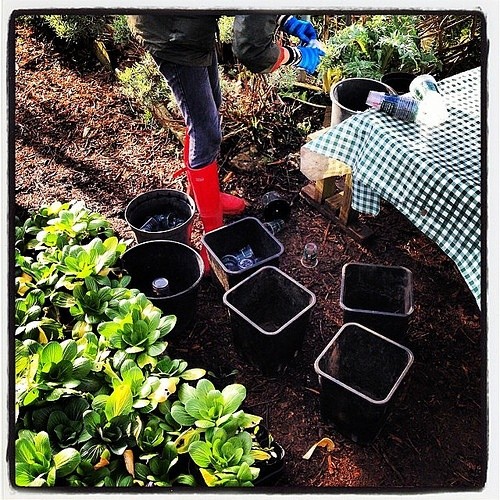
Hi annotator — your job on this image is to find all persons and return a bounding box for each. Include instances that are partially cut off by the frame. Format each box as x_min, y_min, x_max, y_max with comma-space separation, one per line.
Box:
125, 13, 326, 273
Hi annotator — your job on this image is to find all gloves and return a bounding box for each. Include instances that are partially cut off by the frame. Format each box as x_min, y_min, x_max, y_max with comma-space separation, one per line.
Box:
278, 15, 316, 44
296, 46, 325, 75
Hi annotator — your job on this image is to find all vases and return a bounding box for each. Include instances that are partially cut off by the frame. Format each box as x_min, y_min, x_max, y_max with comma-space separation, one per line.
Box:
201, 216, 285, 298
125, 189, 195, 247
312, 322, 414, 423
339, 263, 414, 340
118, 240, 204, 330
222, 265, 316, 375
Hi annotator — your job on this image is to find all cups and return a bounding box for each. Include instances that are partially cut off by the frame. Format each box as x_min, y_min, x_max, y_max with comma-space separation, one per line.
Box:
263, 220, 285, 236
140, 212, 177, 231
300, 243, 319, 268
366, 75, 448, 126
222, 245, 258, 271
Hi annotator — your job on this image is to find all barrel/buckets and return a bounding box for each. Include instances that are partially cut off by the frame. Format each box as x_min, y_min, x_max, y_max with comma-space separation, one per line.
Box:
223, 265, 316, 362
313, 323, 414, 446
329, 77, 397, 127
201, 216, 284, 293
117, 239, 205, 341
340, 262, 414, 340
125, 189, 195, 246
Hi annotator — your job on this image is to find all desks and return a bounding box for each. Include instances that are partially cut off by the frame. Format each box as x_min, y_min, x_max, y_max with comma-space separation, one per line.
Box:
297, 65, 481, 311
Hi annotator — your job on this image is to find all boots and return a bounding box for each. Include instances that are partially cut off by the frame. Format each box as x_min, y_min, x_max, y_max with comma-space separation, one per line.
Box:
173, 130, 246, 215
184, 135, 225, 273
